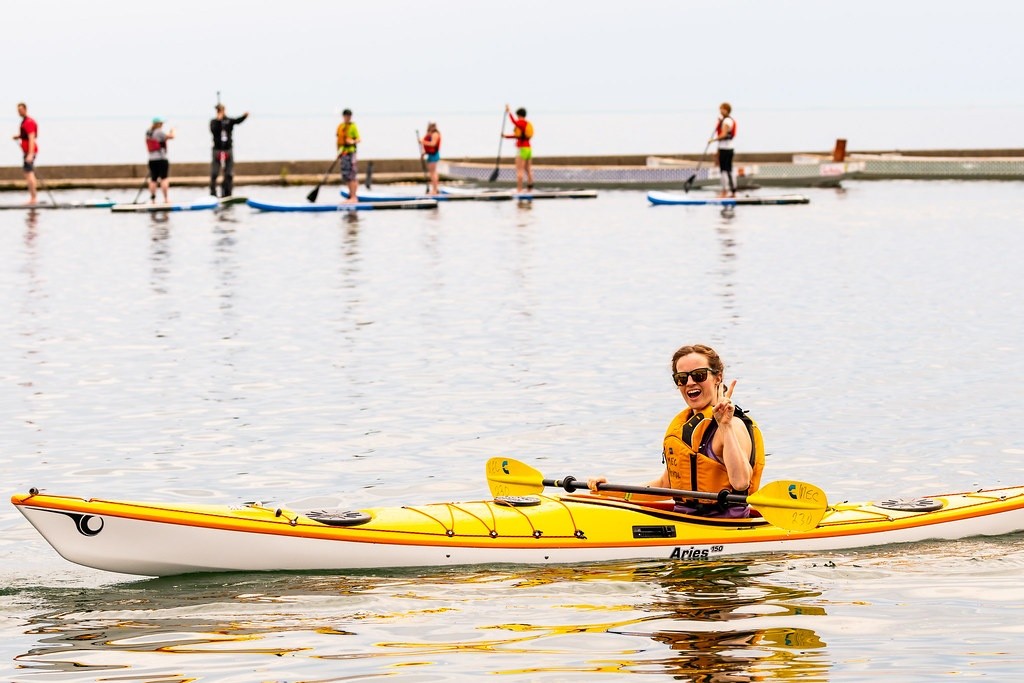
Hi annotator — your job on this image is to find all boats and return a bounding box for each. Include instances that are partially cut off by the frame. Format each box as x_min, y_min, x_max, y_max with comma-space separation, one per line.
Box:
12, 482, 1024, 579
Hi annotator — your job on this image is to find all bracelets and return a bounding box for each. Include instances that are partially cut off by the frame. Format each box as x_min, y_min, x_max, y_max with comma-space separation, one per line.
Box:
625, 492, 632, 501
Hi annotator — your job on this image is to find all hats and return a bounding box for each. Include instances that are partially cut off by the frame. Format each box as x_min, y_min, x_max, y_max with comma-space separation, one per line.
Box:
153, 117, 165, 124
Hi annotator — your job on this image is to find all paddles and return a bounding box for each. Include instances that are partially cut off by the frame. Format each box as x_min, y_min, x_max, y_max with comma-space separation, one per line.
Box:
488, 104, 509, 184
17, 139, 58, 206
216, 91, 232, 198
133, 170, 151, 204
485, 456, 829, 533
682, 127, 718, 194
307, 143, 348, 202
415, 129, 430, 193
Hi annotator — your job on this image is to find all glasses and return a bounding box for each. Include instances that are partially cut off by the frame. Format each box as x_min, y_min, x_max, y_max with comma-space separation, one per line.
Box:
673, 367, 718, 386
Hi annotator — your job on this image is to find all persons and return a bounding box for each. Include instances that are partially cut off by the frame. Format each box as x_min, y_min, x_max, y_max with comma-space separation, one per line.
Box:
708, 103, 736, 199
210, 103, 249, 199
418, 120, 441, 196
501, 104, 534, 193
586, 344, 755, 518
13, 104, 39, 205
336, 110, 359, 204
145, 117, 174, 205
